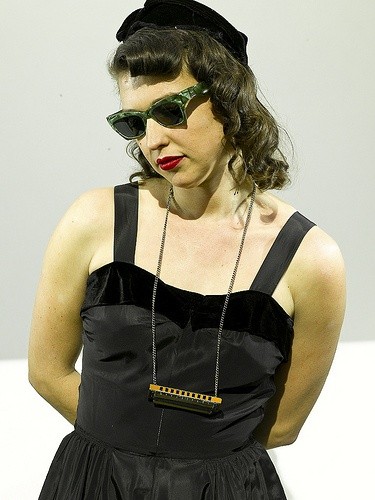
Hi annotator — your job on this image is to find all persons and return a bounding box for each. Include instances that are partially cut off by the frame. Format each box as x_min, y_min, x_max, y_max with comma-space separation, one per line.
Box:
28, 0, 345, 500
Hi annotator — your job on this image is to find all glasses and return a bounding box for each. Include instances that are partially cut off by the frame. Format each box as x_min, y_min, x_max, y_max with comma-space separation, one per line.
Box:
107, 83, 212, 141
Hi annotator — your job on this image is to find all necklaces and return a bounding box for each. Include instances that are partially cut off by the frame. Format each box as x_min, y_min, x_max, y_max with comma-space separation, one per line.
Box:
150, 182, 256, 413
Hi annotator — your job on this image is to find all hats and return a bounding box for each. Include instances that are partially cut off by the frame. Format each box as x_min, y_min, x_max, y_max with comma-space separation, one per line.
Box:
115, 0, 250, 67
